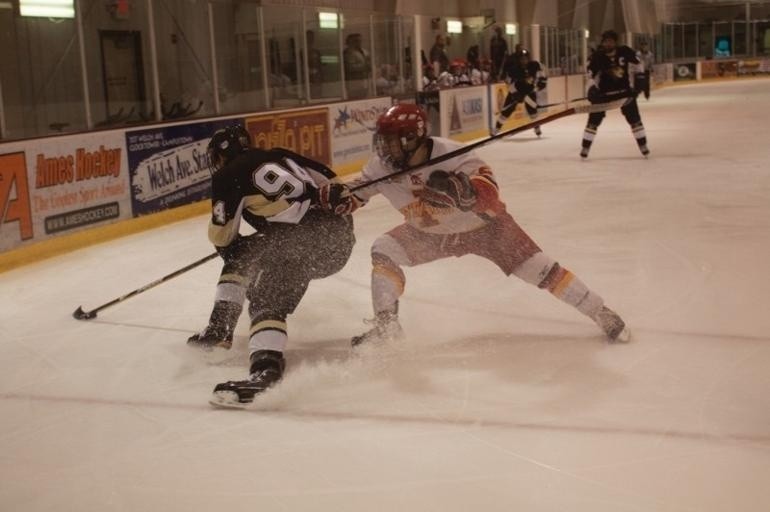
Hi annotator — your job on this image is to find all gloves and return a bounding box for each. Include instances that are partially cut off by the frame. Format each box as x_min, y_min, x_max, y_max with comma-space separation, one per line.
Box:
314, 183, 366, 217
420, 169, 478, 216
587, 84, 598, 101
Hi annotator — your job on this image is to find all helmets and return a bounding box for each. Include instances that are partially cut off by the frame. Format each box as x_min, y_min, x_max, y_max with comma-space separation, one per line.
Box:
600, 29, 618, 42
371, 102, 430, 170
517, 48, 530, 58
202, 119, 254, 180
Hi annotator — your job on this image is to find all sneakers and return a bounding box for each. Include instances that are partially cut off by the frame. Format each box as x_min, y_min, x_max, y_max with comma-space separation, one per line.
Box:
535, 125, 542, 135
590, 305, 628, 345
638, 143, 650, 155
350, 308, 404, 349
579, 146, 589, 158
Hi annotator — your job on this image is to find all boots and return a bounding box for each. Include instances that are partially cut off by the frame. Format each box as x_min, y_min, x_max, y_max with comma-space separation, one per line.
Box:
184, 297, 242, 351
211, 359, 285, 405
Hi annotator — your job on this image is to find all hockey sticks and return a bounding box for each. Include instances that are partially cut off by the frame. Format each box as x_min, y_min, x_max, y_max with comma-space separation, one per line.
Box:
95, 94, 203, 125
352, 88, 639, 192
73, 229, 262, 319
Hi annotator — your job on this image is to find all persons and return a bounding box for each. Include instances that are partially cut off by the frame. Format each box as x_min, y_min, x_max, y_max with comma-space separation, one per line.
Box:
313, 101, 629, 346
634, 41, 655, 101
183, 118, 355, 412
287, 23, 540, 93
488, 47, 549, 140
579, 29, 652, 162
